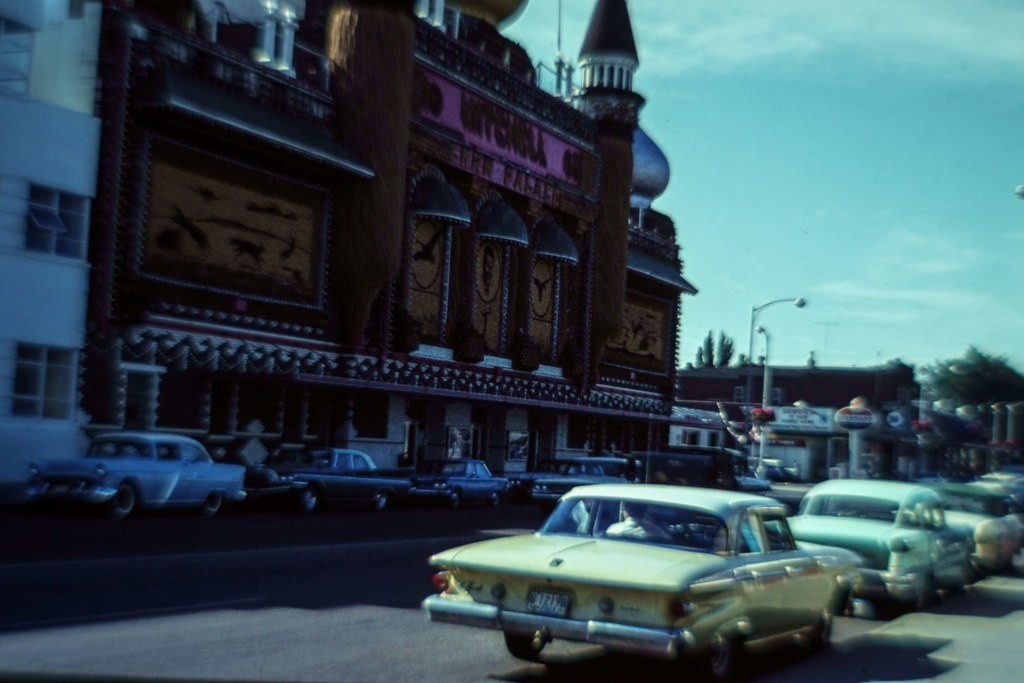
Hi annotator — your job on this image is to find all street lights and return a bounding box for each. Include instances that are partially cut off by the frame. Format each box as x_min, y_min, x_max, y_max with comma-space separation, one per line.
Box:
755, 324, 771, 479
746, 296, 807, 401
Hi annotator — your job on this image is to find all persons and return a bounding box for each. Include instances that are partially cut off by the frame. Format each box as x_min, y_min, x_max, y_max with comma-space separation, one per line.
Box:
604, 500, 674, 541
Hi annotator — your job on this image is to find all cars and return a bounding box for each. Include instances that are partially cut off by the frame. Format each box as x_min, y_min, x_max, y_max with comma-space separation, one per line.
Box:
420, 487, 864, 677
928, 481, 1024, 578
779, 477, 975, 620
402, 457, 508, 510
25, 433, 245, 519
245, 447, 416, 518
529, 457, 646, 507
